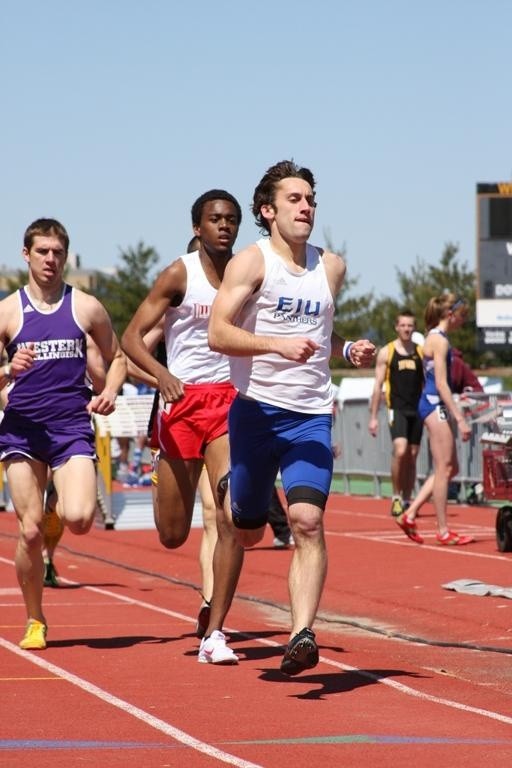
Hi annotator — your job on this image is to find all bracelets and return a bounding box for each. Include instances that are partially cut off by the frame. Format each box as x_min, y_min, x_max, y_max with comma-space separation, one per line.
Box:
7, 362, 12, 377
344, 340, 354, 365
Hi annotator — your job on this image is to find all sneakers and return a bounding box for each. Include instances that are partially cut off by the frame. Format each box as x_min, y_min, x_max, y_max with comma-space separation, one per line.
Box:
18, 619, 48, 649
396, 511, 424, 544
198, 630, 239, 665
280, 628, 318, 676
43, 489, 60, 536
195, 603, 211, 638
435, 532, 474, 546
150, 447, 160, 473
216, 469, 232, 506
273, 533, 296, 545
392, 497, 411, 515
43, 559, 58, 586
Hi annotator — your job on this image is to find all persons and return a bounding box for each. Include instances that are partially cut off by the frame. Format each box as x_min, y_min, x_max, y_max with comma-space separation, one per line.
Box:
370, 310, 426, 518
128, 237, 218, 636
121, 191, 246, 663
266, 481, 296, 549
0, 331, 109, 586
396, 289, 474, 544
0, 217, 130, 648
207, 161, 378, 673
